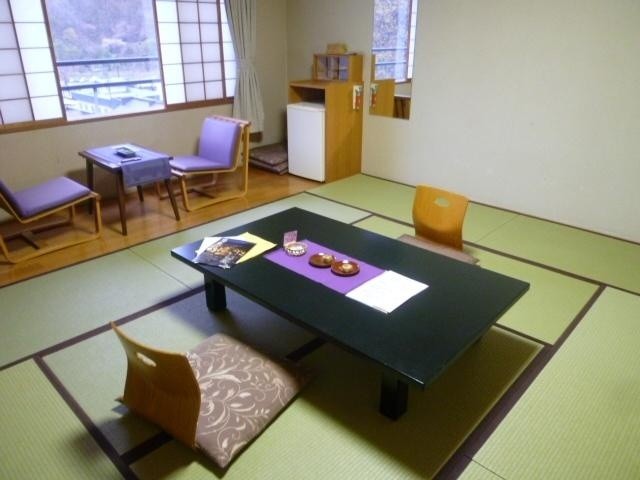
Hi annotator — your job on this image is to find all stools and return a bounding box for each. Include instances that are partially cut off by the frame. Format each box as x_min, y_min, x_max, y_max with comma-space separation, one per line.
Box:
412, 185, 469, 252
109, 319, 202, 453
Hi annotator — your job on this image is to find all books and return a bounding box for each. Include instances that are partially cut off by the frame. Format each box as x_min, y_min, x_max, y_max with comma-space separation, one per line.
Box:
196, 238, 256, 271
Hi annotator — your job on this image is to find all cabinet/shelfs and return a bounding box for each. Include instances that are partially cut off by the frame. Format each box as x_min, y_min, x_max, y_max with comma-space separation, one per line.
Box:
286, 43, 364, 183
370, 54, 395, 118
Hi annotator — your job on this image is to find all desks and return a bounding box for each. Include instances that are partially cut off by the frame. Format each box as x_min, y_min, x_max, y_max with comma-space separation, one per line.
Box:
170, 207, 531, 420
78, 143, 181, 235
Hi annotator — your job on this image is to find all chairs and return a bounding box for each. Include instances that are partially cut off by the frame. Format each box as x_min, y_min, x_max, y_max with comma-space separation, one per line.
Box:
155, 115, 251, 212
0, 176, 104, 265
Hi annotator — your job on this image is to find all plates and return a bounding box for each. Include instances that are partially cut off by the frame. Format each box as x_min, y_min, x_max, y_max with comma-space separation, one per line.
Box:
309, 253, 335, 268
332, 259, 361, 276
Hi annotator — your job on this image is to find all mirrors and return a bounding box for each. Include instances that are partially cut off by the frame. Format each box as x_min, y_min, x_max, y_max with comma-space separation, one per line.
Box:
368, 1, 418, 120
